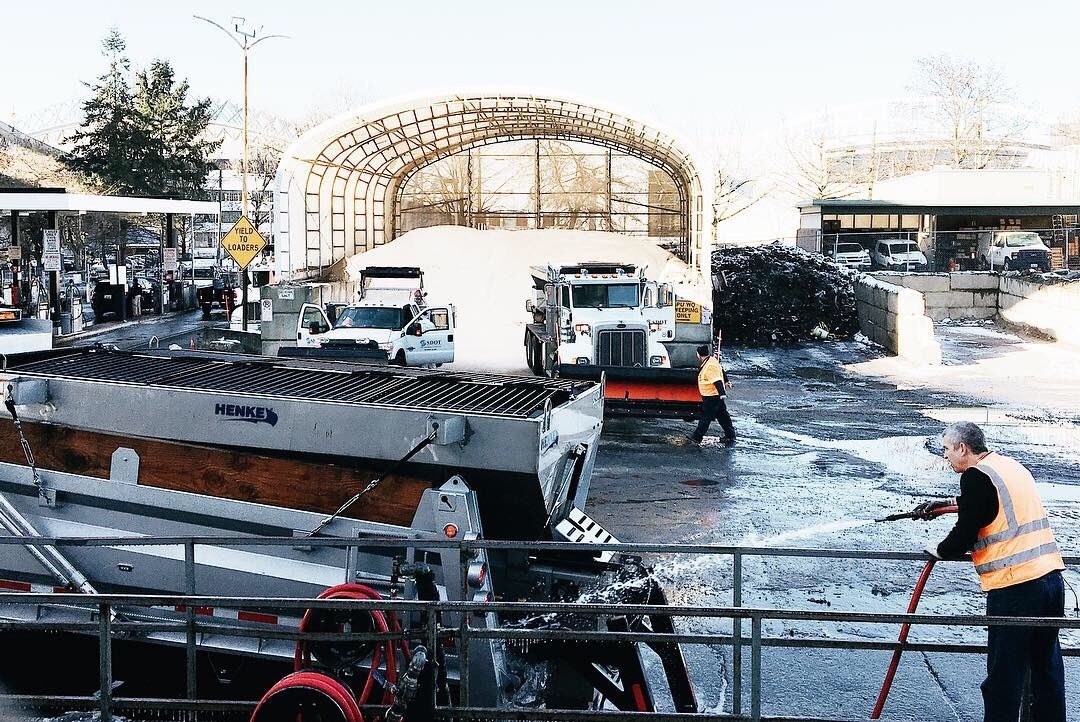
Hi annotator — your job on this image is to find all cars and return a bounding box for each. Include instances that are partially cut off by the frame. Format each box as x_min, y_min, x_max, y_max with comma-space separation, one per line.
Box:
90, 277, 159, 318
60, 281, 87, 300
825, 242, 872, 270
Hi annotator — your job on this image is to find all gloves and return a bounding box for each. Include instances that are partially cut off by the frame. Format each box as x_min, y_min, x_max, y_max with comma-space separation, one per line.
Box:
911, 498, 956, 521
922, 545, 943, 560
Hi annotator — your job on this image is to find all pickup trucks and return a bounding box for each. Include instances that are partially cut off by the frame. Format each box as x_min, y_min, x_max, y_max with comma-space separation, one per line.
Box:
195, 272, 244, 317
867, 238, 928, 274
295, 298, 457, 368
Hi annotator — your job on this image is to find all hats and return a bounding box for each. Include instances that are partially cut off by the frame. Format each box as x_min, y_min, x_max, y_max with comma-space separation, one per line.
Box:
697, 345, 709, 356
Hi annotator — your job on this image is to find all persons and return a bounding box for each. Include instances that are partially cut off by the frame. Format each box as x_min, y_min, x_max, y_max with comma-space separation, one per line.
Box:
131, 277, 142, 316
416, 312, 436, 369
685, 346, 735, 444
913, 420, 1067, 722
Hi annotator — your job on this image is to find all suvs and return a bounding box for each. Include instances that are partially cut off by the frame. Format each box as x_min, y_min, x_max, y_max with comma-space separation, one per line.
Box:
974, 230, 1053, 275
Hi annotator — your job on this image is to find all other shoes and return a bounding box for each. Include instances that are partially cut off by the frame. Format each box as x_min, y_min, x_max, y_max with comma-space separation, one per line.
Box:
719, 436, 735, 443
685, 433, 700, 443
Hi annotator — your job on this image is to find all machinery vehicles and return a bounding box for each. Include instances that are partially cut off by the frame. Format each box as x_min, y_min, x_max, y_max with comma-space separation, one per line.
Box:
521, 260, 719, 423
